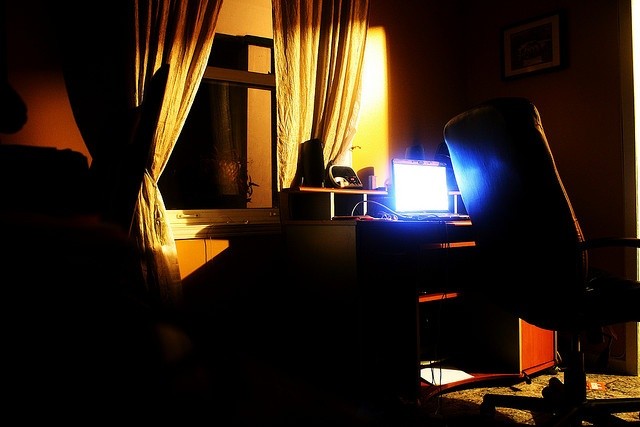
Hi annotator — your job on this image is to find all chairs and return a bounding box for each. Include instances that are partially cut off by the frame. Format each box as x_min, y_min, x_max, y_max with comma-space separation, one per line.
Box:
443, 99, 640, 427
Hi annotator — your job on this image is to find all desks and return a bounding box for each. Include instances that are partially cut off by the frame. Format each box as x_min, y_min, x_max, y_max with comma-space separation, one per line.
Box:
281, 219, 558, 427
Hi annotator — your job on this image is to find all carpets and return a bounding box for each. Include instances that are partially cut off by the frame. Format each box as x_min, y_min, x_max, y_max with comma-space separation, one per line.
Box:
419, 373, 640, 427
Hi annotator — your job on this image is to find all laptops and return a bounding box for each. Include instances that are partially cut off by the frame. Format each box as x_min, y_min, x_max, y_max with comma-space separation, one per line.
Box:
393, 158, 469, 223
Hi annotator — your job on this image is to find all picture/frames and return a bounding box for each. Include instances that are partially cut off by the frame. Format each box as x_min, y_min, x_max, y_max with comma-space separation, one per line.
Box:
498, 14, 570, 82
